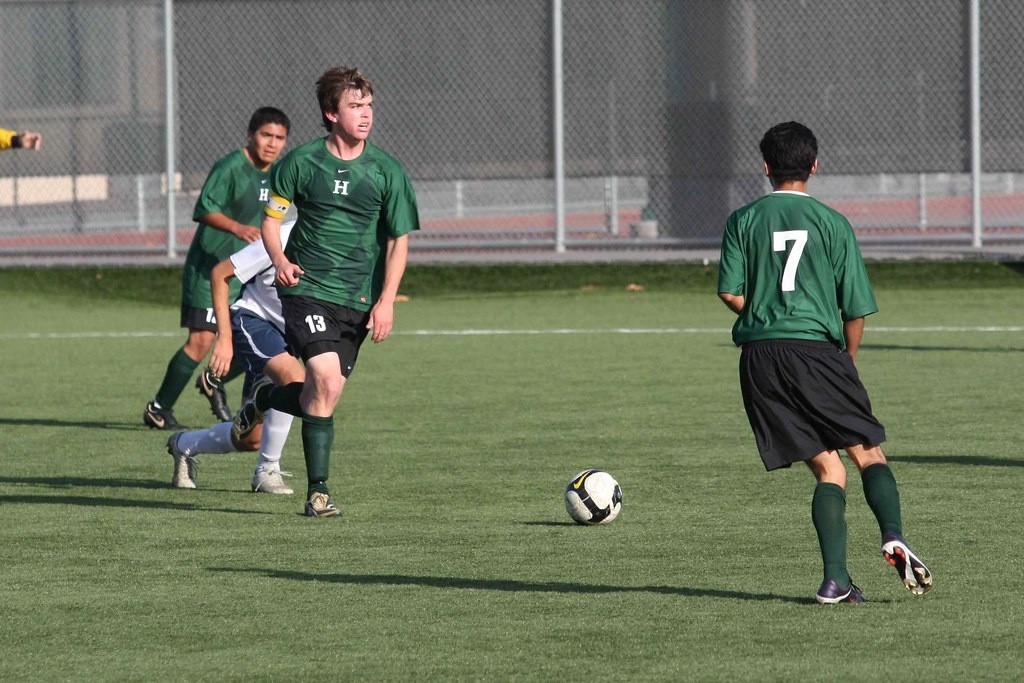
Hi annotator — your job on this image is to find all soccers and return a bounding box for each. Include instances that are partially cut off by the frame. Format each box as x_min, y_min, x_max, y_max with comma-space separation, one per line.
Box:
565, 468, 622, 525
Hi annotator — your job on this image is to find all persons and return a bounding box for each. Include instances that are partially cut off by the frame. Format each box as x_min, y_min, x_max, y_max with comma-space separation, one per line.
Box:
1, 119, 44, 157
237, 63, 423, 521
141, 105, 293, 433
164, 217, 310, 496
714, 121, 934, 603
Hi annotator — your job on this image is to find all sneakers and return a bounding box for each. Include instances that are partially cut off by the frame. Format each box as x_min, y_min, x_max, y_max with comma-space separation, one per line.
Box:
304, 491, 343, 518
250, 467, 294, 495
143, 401, 190, 431
195, 368, 233, 422
881, 532, 934, 595
166, 430, 197, 489
815, 578, 864, 604
